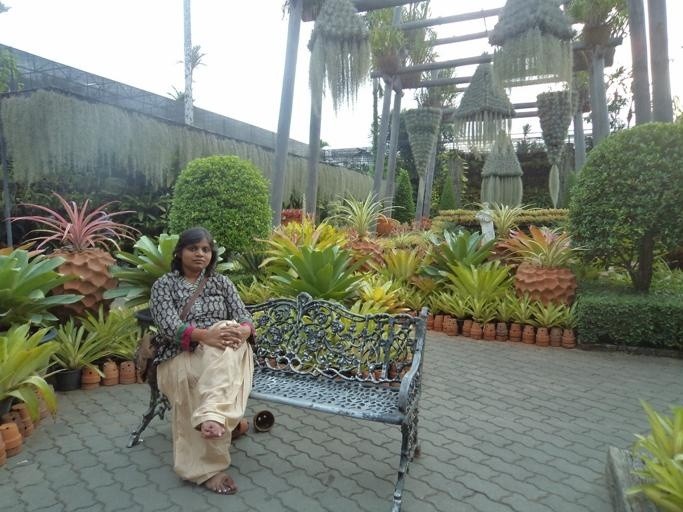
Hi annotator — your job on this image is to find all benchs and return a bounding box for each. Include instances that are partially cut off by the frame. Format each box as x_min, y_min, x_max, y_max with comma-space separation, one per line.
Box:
127, 290, 432, 512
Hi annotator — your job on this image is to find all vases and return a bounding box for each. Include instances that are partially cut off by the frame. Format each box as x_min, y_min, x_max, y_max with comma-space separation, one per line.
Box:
229, 409, 274, 439
0, 359, 147, 466
406, 309, 577, 348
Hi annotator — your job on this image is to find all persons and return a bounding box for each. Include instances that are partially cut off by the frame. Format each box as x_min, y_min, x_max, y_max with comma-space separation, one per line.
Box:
148, 226, 257, 496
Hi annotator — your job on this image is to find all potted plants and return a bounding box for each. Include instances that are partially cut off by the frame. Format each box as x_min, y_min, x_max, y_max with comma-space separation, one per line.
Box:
3, 189, 146, 322
99, 233, 225, 335
494, 224, 589, 311
323, 187, 405, 274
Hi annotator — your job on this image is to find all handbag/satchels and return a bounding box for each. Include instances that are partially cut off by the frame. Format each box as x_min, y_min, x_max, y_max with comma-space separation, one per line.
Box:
137, 332, 155, 382
